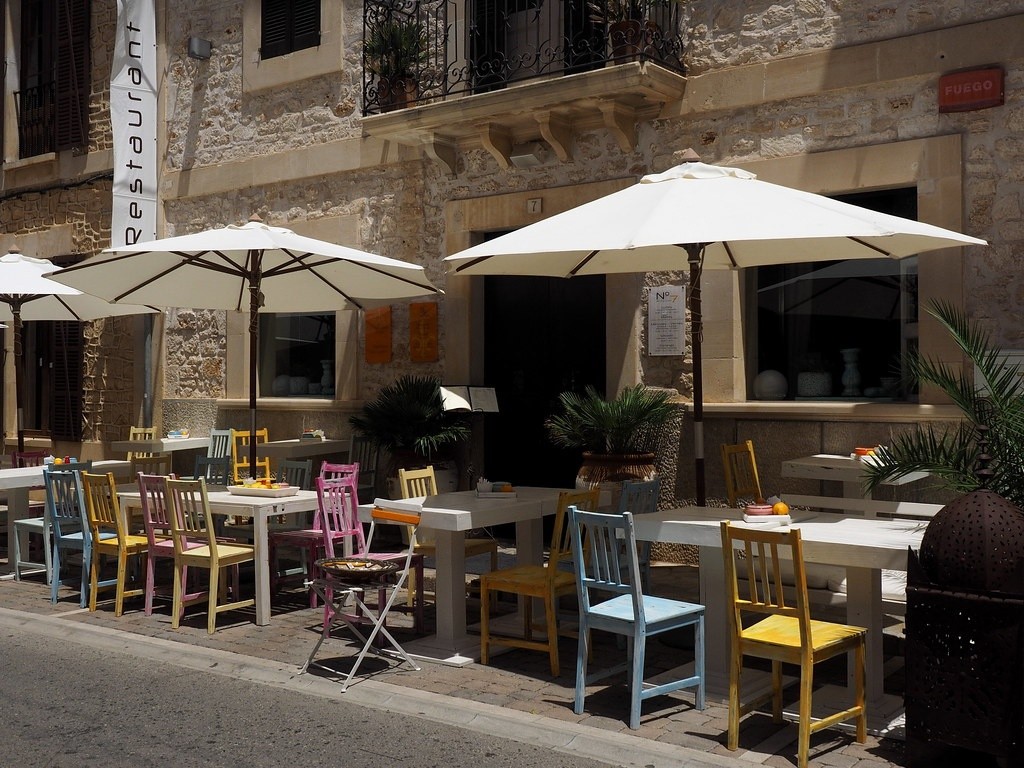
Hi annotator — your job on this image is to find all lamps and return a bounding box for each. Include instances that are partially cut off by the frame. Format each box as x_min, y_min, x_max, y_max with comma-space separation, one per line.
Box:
188, 37, 212, 61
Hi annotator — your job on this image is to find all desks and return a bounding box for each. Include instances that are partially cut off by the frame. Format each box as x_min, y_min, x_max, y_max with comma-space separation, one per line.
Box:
64, 459, 132, 568
70, 485, 353, 626
442, 487, 611, 642
0, 458, 62, 581
751, 514, 930, 742
239, 436, 351, 485
110, 433, 211, 475
615, 505, 817, 709
357, 486, 542, 667
782, 453, 932, 516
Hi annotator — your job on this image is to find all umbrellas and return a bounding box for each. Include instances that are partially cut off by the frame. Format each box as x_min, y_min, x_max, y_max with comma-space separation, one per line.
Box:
40, 215, 446, 480
441, 147, 989, 505
0, 246, 166, 469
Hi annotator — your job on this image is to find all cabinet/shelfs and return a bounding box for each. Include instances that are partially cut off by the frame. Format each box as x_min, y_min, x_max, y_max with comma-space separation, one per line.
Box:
900, 256, 918, 402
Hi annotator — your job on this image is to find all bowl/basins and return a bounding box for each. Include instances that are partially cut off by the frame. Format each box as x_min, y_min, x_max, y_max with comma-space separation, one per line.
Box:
855, 448, 873, 456
274, 483, 289, 488
493, 482, 513, 492
746, 505, 773, 516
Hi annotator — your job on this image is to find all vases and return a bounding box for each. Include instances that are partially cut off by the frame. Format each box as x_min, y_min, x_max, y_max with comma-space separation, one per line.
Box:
839, 347, 864, 396
320, 360, 335, 395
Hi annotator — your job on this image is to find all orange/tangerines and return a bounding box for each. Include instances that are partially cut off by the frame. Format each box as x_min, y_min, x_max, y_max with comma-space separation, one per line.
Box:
773, 502, 789, 515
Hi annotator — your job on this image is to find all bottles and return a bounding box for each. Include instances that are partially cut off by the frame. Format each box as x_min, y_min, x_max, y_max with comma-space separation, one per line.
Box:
767, 494, 781, 506
841, 350, 862, 397
65, 455, 70, 464
44, 455, 55, 465
758, 498, 764, 505
69, 458, 77, 464
243, 477, 256, 486
874, 444, 888, 456
55, 458, 62, 464
170, 429, 188, 435
477, 478, 492, 493
305, 429, 324, 437
62, 457, 65, 464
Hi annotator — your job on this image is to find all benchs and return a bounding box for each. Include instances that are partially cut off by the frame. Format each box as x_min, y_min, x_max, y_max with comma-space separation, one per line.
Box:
735, 494, 947, 616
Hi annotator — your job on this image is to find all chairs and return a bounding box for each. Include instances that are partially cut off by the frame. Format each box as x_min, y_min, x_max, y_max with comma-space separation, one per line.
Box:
310, 433, 381, 504
179, 426, 233, 486
568, 505, 707, 731
162, 475, 257, 634
193, 454, 230, 488
13, 460, 92, 587
720, 439, 762, 506
137, 472, 243, 623
42, 467, 141, 609
129, 454, 172, 482
80, 470, 168, 617
554, 475, 660, 650
316, 474, 424, 647
478, 486, 602, 678
127, 425, 157, 461
301, 498, 423, 693
270, 460, 360, 610
230, 427, 271, 486
222, 458, 312, 590
719, 518, 870, 768
398, 465, 497, 617
11, 450, 50, 561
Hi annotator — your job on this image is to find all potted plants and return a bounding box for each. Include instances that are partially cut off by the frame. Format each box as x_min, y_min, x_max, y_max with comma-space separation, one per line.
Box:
588, 0, 672, 67
344, 375, 479, 547
544, 384, 678, 515
866, 296, 1024, 592
363, 23, 430, 114
